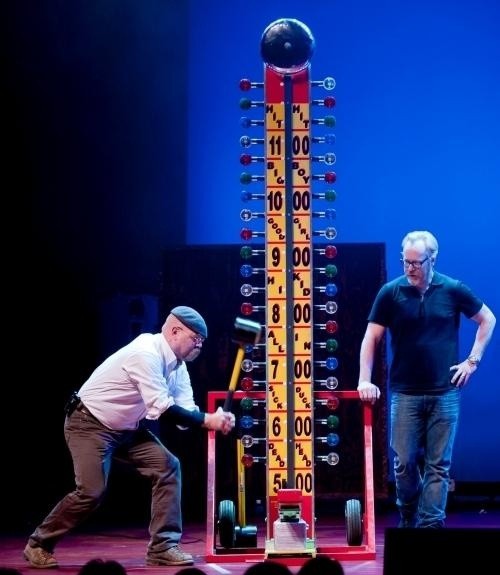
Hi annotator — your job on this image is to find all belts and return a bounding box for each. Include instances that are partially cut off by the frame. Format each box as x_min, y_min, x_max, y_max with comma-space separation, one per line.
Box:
77, 402, 91, 416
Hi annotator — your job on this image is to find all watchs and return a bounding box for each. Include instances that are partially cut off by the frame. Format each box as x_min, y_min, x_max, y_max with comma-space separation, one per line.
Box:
467, 354, 480, 366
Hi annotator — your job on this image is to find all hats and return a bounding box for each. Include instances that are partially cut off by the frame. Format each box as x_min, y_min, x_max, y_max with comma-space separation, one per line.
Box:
170, 305, 208, 339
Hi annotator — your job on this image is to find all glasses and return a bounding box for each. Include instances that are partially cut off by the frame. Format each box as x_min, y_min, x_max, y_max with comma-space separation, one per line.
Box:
177, 326, 206, 343
401, 259, 429, 269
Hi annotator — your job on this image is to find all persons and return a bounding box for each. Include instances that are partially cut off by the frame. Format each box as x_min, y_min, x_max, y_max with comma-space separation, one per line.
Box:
21, 304, 239, 566
79, 558, 127, 575
245, 555, 344, 575
356, 228, 498, 530
176, 568, 205, 575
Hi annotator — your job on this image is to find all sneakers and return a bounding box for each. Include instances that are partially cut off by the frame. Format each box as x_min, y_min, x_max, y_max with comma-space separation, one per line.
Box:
146, 547, 195, 566
23, 542, 60, 568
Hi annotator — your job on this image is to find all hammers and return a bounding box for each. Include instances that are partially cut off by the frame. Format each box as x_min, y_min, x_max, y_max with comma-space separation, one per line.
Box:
223, 335, 254, 412
